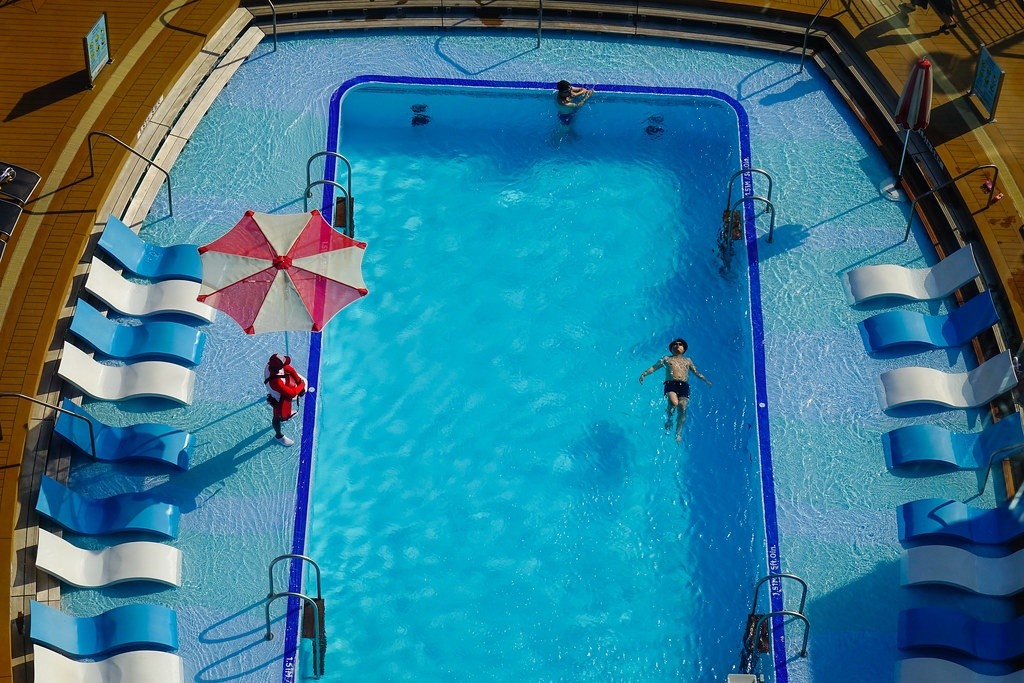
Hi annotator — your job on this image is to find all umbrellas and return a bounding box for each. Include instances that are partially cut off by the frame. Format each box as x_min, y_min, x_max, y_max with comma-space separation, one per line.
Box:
196, 208, 370, 366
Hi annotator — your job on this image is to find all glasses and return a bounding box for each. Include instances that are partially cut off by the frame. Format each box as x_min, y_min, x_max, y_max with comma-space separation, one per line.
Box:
674, 343, 683, 346
562, 87, 572, 94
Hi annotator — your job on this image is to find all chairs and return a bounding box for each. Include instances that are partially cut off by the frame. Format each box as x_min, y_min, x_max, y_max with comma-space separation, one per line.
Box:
895, 480, 1024, 541
843, 242, 980, 307
33, 527, 186, 590
877, 349, 1019, 415
55, 340, 199, 408
892, 656, 1024, 682
900, 544, 1024, 596
52, 397, 197, 473
855, 286, 1002, 358
896, 606, 1024, 654
29, 596, 182, 656
84, 256, 222, 325
31, 642, 185, 683
879, 411, 1024, 472
96, 213, 228, 292
33, 474, 182, 540
67, 297, 209, 367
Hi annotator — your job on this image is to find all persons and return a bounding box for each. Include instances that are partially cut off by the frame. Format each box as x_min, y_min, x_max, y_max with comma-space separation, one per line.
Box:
556, 80, 594, 141
263, 353, 304, 448
639, 338, 714, 440
718, 211, 741, 272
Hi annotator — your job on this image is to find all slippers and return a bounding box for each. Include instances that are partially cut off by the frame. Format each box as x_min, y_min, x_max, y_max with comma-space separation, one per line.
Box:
273, 434, 294, 447
281, 410, 298, 423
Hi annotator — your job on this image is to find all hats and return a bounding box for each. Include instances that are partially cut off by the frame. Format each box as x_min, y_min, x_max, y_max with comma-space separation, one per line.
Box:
268, 353, 291, 367
